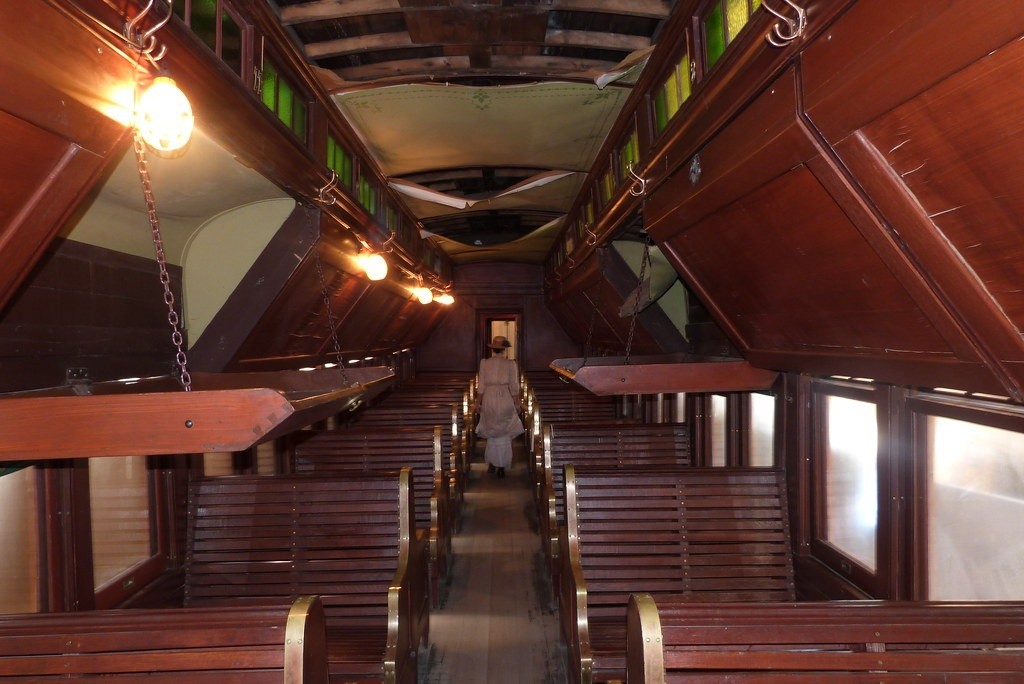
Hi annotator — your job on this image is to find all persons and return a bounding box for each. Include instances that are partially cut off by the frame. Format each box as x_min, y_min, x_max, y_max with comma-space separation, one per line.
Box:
475, 336, 524, 478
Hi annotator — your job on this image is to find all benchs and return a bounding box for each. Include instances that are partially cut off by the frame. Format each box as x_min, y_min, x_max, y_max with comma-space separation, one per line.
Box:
0, 368, 480, 683
521, 370, 1024, 683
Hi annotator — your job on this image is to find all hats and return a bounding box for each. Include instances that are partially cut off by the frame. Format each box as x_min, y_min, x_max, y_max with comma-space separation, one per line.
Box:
487, 336, 512, 348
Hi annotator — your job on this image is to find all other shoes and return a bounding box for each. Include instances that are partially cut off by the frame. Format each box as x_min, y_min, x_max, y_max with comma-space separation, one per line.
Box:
497, 468, 505, 477
488, 463, 495, 472
